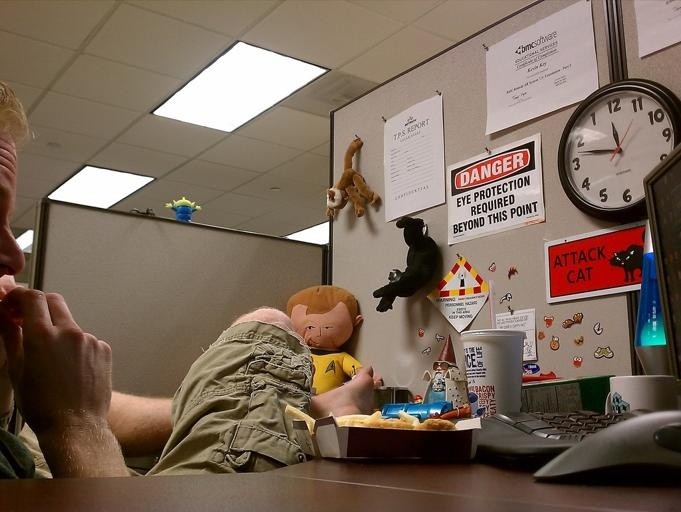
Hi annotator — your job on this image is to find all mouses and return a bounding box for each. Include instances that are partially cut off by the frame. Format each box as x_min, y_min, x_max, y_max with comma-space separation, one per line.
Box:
533, 409, 681, 489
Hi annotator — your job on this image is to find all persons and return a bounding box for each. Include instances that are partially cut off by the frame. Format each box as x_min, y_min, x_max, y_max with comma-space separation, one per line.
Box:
1, 78, 383, 481
283, 286, 382, 398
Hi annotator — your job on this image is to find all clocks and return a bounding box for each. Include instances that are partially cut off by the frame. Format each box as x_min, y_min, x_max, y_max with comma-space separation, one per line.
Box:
557, 78, 681, 224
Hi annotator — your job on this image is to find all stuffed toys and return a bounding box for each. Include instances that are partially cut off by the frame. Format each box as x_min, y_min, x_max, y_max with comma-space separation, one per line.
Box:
371, 215, 441, 312
324, 138, 379, 219
163, 196, 203, 222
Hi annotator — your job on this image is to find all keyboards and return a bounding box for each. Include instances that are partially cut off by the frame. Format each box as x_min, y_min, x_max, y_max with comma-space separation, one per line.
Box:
477, 411, 639, 474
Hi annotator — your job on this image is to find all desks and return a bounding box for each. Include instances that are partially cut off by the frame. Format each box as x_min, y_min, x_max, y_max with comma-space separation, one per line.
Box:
0, 456, 681, 512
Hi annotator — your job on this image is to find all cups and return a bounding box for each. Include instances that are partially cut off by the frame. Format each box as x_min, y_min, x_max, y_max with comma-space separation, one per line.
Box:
460, 328, 526, 421
609, 373, 681, 416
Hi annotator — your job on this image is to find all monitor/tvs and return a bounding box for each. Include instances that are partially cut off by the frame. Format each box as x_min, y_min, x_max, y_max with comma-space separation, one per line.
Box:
643, 144, 681, 388
